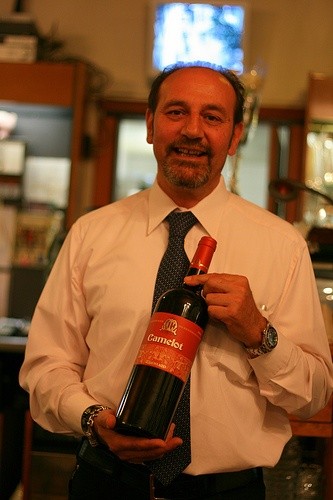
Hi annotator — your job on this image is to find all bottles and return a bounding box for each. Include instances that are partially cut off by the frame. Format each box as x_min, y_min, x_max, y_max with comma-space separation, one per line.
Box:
114, 236, 217, 441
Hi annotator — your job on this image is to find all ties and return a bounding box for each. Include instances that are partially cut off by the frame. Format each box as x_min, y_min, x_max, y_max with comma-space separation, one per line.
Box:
145, 208, 197, 489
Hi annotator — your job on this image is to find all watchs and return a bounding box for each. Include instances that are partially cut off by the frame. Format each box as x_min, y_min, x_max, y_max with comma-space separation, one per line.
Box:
242, 317, 278, 357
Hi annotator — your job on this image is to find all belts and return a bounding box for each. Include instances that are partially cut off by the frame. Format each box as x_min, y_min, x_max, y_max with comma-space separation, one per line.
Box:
80, 442, 260, 499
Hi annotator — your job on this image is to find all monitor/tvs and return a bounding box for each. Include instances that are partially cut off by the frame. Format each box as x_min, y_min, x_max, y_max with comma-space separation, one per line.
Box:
145, 0, 250, 80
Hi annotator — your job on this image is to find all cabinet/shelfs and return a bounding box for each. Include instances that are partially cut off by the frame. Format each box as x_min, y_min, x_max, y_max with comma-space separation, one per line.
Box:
1, 61, 332, 500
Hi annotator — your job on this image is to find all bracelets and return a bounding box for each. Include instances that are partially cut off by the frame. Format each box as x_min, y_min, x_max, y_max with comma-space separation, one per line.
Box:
81, 405, 111, 447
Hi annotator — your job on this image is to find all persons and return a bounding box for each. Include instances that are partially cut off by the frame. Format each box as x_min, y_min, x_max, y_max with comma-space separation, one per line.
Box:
19, 61, 333, 500
